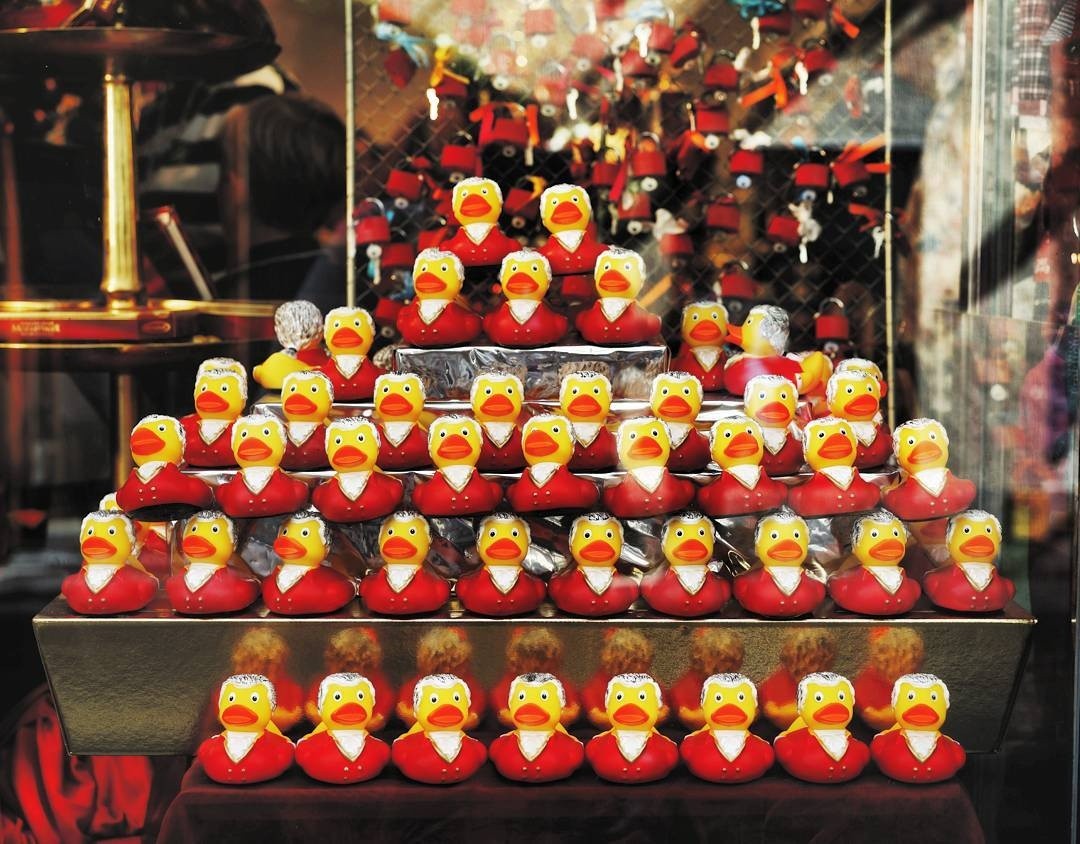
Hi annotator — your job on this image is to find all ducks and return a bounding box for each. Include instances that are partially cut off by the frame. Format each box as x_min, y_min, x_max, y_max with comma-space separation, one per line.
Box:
61, 177, 1017, 784
